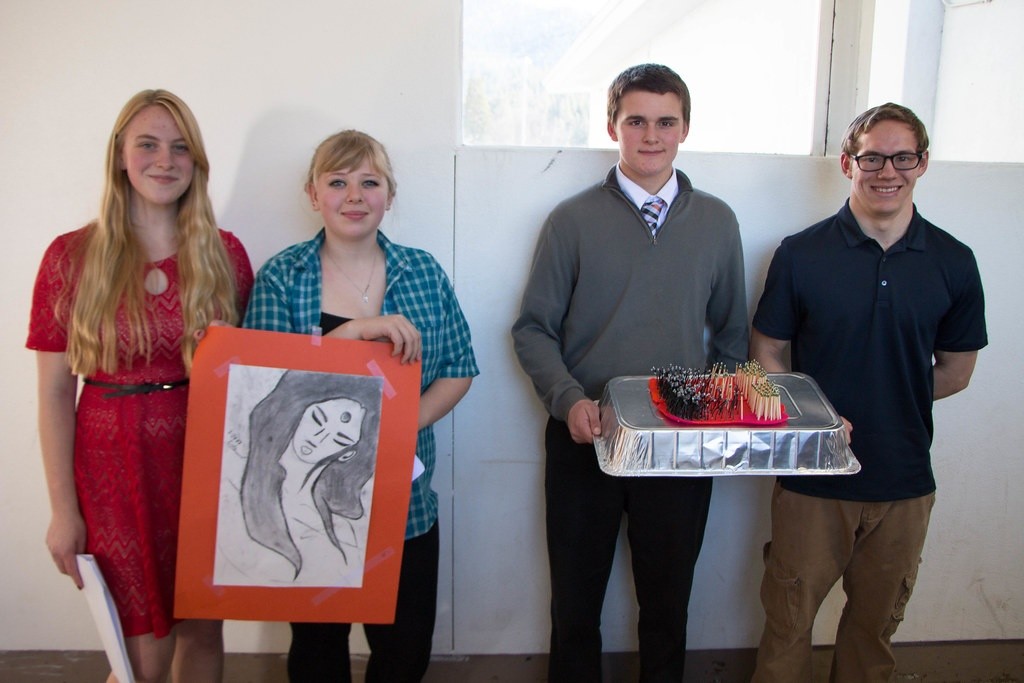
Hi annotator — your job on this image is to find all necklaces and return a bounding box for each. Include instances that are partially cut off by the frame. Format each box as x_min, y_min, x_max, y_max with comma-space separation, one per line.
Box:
320, 244, 380, 305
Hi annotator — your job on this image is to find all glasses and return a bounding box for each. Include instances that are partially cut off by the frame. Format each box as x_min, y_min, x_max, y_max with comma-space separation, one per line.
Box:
850, 152, 923, 172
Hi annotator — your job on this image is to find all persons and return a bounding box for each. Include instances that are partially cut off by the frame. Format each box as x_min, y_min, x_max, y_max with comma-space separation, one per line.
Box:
244, 129, 479, 683
25, 88, 255, 683
510, 63, 750, 683
751, 103, 988, 682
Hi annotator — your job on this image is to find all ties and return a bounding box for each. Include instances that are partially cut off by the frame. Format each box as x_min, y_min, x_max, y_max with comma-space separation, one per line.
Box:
641, 198, 665, 240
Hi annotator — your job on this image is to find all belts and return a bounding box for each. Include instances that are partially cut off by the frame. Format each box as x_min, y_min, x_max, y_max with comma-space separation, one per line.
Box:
83, 378, 190, 399
